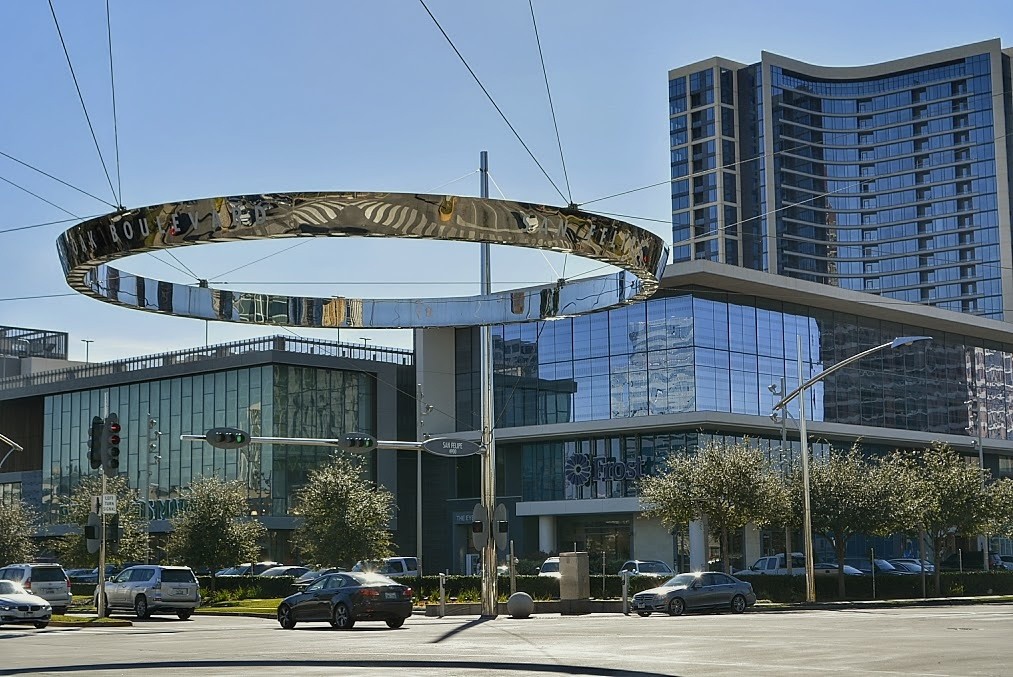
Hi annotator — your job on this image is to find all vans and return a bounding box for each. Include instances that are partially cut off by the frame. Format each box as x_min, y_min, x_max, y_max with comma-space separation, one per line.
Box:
351, 556, 419, 579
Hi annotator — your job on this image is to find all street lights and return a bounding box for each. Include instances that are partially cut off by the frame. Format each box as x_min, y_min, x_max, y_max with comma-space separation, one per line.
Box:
80, 339, 94, 366
772, 333, 933, 612
144, 413, 164, 566
767, 376, 793, 577
964, 397, 989, 571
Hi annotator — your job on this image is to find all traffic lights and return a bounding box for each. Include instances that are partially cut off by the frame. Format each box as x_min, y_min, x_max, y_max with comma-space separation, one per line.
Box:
338, 432, 378, 453
206, 427, 251, 450
87, 416, 104, 469
470, 502, 489, 551
100, 412, 121, 481
109, 513, 128, 557
84, 512, 99, 554
493, 503, 510, 551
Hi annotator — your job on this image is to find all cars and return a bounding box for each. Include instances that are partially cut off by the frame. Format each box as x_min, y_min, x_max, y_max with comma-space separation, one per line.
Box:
293, 567, 347, 592
208, 567, 231, 576
260, 565, 311, 579
998, 555, 1013, 571
0, 580, 54, 628
813, 563, 865, 578
276, 571, 413, 631
66, 569, 94, 577
12, 338, 31, 353
631, 573, 758, 618
618, 559, 674, 577
842, 558, 936, 574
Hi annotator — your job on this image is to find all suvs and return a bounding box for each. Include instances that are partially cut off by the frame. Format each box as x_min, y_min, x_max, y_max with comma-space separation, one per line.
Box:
535, 557, 561, 580
730, 552, 806, 576
940, 550, 1006, 571
224, 561, 284, 576
93, 565, 202, 621
0, 563, 72, 615
70, 562, 145, 596
